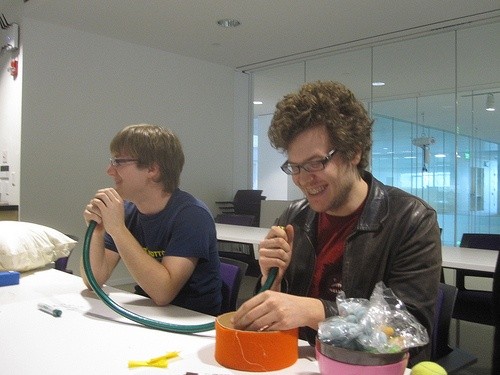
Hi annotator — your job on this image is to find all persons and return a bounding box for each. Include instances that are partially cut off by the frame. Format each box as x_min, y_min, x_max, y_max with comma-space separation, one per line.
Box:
230, 79, 442, 369
79, 124, 223, 317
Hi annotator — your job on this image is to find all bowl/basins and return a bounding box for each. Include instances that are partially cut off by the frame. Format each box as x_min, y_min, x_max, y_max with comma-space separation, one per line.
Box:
315, 335, 405, 366
315, 347, 409, 375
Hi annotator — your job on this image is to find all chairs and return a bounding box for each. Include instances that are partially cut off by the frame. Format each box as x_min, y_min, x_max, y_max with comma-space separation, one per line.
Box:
211, 189, 265, 228
218, 258, 249, 312
432, 234, 500, 375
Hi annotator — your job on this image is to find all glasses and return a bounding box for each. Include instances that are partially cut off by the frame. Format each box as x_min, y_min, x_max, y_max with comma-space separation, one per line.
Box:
281, 149, 338, 175
107, 158, 147, 168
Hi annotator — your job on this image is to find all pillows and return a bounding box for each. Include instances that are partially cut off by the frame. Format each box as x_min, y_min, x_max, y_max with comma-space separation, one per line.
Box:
0, 221, 80, 274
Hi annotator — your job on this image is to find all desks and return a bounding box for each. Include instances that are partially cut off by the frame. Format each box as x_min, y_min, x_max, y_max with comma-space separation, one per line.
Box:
214, 221, 269, 243
440, 245, 499, 274
0, 268, 414, 375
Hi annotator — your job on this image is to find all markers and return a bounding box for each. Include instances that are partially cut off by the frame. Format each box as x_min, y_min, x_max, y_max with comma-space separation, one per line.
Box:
36, 301, 63, 318
